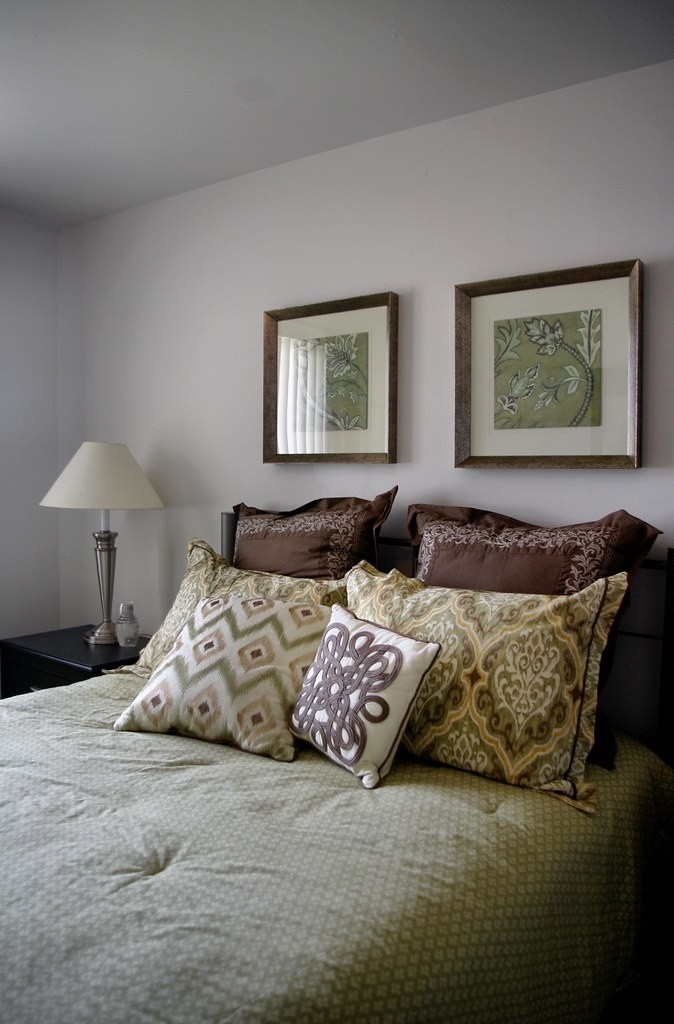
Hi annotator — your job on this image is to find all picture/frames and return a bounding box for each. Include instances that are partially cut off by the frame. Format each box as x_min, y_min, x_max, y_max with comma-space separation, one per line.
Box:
263, 291, 400, 465
453, 259, 642, 471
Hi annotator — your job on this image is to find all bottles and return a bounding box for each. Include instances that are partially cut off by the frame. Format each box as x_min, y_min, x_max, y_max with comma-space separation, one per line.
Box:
116, 601, 139, 648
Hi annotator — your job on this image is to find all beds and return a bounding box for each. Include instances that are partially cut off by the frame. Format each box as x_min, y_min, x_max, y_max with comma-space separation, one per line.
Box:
1, 512, 674, 1024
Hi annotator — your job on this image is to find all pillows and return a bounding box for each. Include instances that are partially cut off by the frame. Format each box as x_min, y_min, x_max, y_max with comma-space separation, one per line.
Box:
344, 560, 629, 813
115, 597, 331, 762
290, 602, 444, 790
102, 535, 348, 680
406, 504, 664, 693
233, 485, 399, 580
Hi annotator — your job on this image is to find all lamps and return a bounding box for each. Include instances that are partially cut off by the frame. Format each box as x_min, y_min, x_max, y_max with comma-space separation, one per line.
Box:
39, 441, 165, 645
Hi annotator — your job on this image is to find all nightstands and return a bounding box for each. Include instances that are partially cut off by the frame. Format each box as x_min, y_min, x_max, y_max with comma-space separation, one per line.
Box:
0, 624, 153, 700
